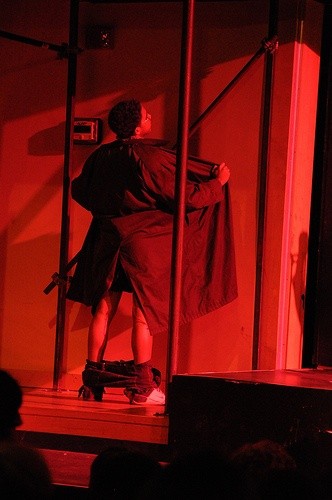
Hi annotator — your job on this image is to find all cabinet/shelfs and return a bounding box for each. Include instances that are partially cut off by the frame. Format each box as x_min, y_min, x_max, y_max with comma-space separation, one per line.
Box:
164, 365, 332, 500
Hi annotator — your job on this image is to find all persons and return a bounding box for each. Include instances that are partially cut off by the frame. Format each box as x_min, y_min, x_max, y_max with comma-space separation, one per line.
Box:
69, 97, 231, 405
0, 367, 331, 500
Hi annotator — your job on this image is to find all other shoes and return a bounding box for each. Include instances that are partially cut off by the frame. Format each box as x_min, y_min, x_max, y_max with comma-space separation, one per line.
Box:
133, 386, 167, 406
82, 385, 102, 399
